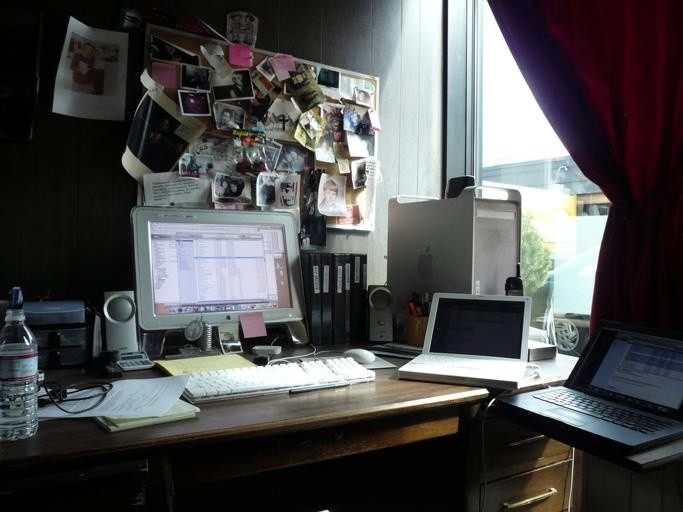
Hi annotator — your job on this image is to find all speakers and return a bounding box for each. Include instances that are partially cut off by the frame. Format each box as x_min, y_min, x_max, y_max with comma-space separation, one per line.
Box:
103, 291, 138, 354
367, 284, 394, 343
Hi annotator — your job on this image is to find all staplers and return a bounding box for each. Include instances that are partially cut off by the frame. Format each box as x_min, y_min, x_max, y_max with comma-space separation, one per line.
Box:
82, 351, 121, 379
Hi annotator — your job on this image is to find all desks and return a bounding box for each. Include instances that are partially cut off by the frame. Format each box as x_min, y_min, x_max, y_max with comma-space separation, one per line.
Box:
0, 339, 584, 510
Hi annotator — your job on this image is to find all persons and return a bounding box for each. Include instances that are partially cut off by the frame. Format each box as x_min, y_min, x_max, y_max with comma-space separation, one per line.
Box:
69, 41, 105, 96
216, 108, 240, 130
229, 70, 249, 98
203, 162, 215, 178
185, 155, 202, 178
319, 179, 345, 216
259, 175, 275, 205
278, 181, 297, 206
213, 174, 244, 199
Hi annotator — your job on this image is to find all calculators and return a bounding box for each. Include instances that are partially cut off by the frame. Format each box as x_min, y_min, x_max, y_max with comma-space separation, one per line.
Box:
117, 352, 155, 372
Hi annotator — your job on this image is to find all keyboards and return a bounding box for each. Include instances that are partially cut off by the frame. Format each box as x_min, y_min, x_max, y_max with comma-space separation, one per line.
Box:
172, 357, 376, 404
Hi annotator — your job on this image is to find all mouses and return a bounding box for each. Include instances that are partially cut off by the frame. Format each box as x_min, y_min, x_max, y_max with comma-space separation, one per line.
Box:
344, 348, 377, 364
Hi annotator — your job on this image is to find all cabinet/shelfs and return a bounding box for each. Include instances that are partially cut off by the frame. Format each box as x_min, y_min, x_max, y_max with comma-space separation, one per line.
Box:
457, 404, 583, 511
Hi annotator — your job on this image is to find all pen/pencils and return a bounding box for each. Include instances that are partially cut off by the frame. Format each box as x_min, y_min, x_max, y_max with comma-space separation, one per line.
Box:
408, 291, 433, 317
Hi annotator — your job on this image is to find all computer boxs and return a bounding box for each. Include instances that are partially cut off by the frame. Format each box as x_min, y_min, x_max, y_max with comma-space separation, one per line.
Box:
387, 185, 521, 344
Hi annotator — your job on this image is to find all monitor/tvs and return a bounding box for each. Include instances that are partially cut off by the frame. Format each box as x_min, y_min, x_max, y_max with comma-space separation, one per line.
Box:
130, 205, 307, 361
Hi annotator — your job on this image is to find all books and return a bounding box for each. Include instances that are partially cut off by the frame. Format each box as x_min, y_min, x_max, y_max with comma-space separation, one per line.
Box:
151, 352, 261, 376
92, 411, 196, 433
102, 398, 200, 427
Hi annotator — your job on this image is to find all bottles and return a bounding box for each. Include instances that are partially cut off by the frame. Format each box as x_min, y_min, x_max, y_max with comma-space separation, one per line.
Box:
0, 309, 38, 441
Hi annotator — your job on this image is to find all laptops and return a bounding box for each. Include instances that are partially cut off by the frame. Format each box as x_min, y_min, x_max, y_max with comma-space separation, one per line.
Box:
495, 319, 683, 455
397, 293, 532, 391
